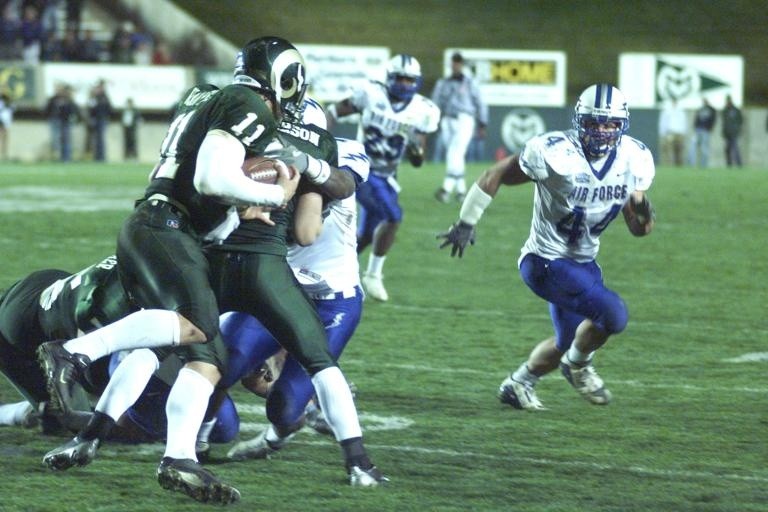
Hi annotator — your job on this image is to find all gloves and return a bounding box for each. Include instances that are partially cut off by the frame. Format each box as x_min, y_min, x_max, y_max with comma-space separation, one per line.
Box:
631, 194, 654, 233
436, 219, 475, 258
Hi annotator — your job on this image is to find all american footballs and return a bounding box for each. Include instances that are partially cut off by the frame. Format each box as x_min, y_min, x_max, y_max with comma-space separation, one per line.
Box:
214, 157, 295, 207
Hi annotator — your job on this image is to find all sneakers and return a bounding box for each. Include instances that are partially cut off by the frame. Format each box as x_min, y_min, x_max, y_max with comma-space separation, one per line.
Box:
362, 272, 388, 302
157, 456, 241, 508
559, 350, 613, 405
36, 340, 99, 473
347, 464, 391, 488
195, 402, 334, 459
497, 376, 550, 412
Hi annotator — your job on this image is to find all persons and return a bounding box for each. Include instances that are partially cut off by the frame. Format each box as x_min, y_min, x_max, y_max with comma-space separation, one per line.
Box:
499, 107, 545, 152
688, 98, 717, 171
660, 94, 689, 167
1, 1, 220, 66
430, 82, 657, 417
722, 92, 745, 168
428, 51, 492, 204
323, 54, 441, 304
0, 36, 388, 502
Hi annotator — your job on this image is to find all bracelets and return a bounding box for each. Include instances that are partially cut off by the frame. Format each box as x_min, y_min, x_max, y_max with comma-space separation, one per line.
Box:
454, 181, 492, 231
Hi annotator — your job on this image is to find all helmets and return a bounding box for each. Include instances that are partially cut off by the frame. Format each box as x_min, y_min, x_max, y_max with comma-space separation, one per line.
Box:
233, 36, 328, 130
573, 83, 630, 158
385, 54, 423, 100
